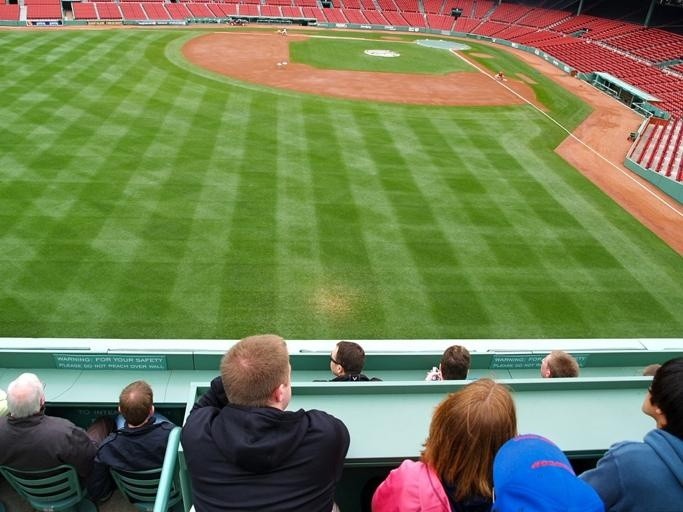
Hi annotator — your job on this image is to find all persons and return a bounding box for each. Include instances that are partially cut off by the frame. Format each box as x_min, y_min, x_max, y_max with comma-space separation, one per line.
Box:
0, 371, 119, 506
310, 337, 385, 382
574, 354, 682, 512
356, 377, 519, 512
537, 348, 580, 380
91, 379, 180, 505
181, 333, 351, 512
423, 343, 471, 380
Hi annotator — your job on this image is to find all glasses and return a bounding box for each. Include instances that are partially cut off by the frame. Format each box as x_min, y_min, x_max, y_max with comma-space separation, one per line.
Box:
327, 354, 339, 365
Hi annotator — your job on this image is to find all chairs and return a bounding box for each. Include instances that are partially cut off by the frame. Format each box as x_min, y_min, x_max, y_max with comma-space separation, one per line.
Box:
0, 464, 181, 512
0, 0, 525, 46
524, 2, 681, 186
